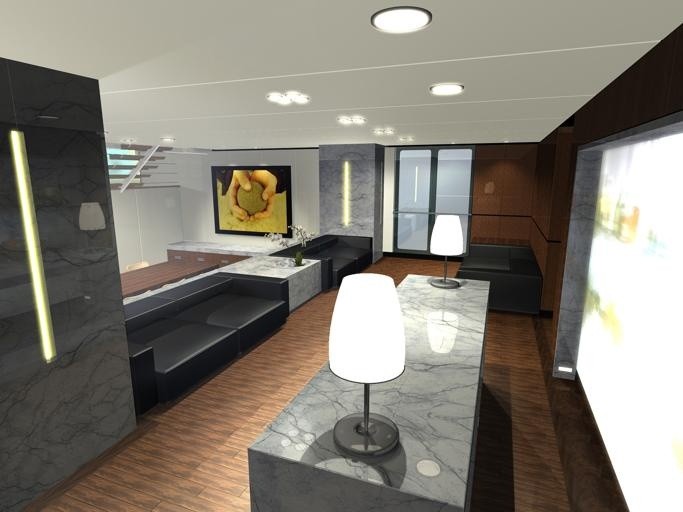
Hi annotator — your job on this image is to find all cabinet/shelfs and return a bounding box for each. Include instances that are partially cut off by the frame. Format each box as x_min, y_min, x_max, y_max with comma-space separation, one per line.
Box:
166, 250, 251, 266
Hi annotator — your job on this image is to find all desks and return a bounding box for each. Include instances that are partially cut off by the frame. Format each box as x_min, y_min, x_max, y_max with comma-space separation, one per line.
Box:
247, 274, 491, 511
120, 258, 219, 299
218, 254, 322, 313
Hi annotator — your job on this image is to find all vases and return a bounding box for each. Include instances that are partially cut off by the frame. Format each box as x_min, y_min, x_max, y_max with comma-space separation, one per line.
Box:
295, 252, 303, 265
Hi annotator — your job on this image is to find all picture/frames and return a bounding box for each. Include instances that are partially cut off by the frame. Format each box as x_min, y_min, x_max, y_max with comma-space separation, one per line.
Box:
210, 165, 293, 239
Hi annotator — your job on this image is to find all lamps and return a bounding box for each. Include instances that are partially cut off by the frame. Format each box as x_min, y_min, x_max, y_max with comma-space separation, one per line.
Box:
78, 200, 106, 255
327, 271, 406, 459
429, 214, 465, 290
427, 289, 460, 354
370, 5, 433, 34
428, 83, 464, 96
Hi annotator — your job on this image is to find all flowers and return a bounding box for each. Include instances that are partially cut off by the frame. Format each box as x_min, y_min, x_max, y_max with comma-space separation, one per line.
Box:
263, 224, 317, 253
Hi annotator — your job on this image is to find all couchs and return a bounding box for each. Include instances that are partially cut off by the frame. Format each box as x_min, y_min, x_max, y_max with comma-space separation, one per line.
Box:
123, 273, 289, 414
269, 235, 373, 291
454, 242, 543, 317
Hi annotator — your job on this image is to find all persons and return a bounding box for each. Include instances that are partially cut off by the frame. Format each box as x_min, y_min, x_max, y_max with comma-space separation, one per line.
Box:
226, 170, 277, 221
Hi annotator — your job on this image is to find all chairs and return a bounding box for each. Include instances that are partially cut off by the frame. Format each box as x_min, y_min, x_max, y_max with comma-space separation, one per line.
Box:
125, 261, 149, 273
162, 278, 185, 288
123, 289, 151, 301
